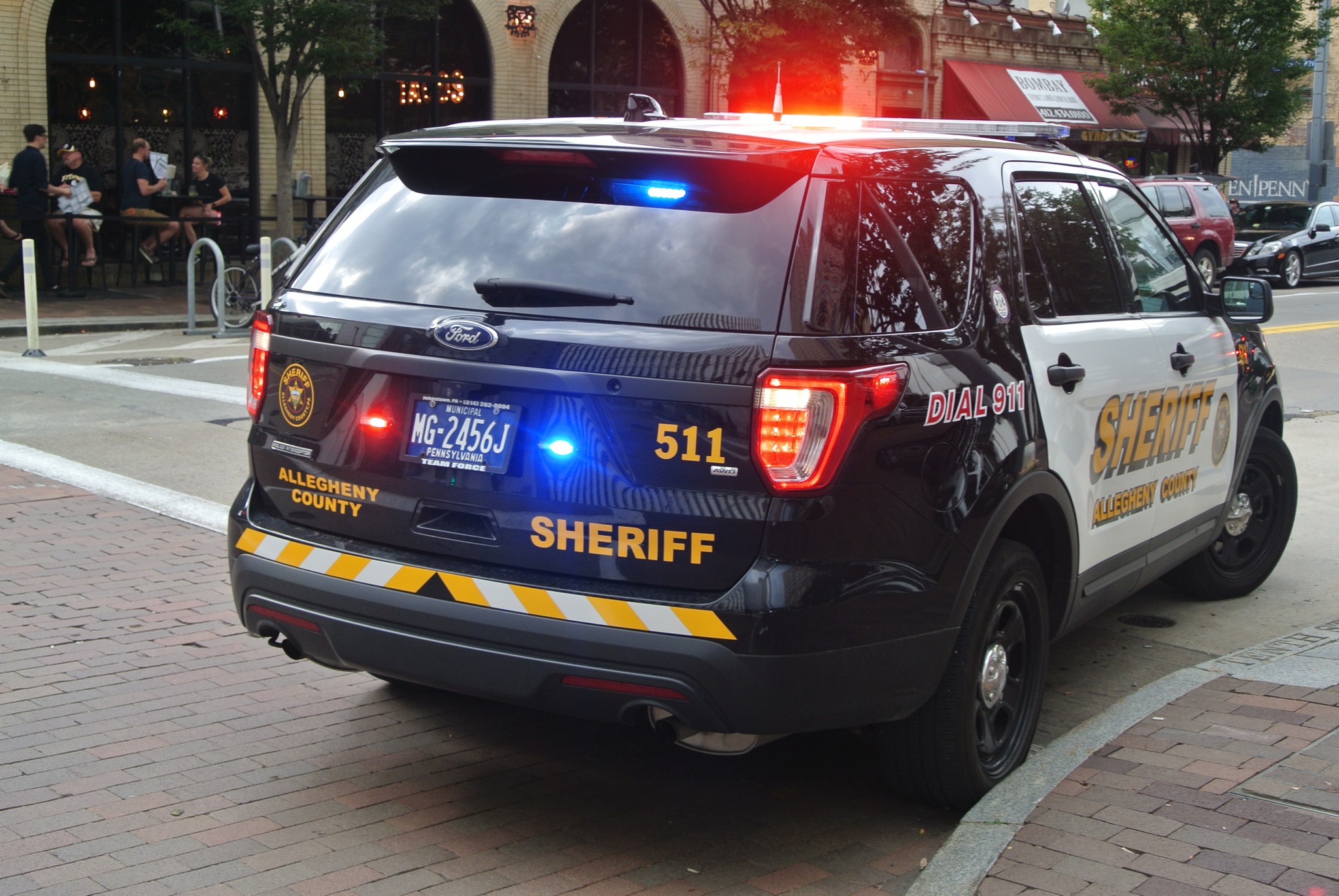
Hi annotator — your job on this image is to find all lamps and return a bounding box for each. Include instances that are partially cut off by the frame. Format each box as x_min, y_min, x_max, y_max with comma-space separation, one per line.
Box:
1006, 15, 1022, 32
504, 4, 538, 38
1047, 20, 1062, 37
963, 9, 980, 27
1086, 24, 1101, 38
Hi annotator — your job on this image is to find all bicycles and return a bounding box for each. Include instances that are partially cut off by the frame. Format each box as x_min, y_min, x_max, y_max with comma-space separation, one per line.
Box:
209, 241, 292, 331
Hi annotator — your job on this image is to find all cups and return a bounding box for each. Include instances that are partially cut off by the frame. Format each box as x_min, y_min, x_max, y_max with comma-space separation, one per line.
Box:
189, 185, 195, 195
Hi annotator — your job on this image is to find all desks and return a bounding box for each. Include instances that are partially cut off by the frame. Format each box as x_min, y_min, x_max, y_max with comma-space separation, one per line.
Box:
272, 192, 344, 240
151, 195, 248, 281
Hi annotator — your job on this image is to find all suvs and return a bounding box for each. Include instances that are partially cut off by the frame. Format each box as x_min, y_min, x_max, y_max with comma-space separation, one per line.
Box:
1124, 174, 1235, 292
226, 91, 1301, 808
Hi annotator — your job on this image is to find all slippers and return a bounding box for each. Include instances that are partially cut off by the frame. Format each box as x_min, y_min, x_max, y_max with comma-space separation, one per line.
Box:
60, 257, 69, 267
3, 231, 23, 241
81, 255, 99, 267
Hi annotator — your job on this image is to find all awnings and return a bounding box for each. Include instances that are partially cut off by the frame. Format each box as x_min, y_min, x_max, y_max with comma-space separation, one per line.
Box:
940, 57, 1261, 148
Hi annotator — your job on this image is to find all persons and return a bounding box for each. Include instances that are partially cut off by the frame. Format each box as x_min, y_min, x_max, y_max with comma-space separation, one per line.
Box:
1229, 198, 1244, 218
118, 138, 180, 264
0, 123, 70, 298
0, 142, 106, 269
179, 154, 233, 264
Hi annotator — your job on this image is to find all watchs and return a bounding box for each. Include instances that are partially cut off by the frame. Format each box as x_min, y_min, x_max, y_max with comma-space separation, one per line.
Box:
212, 202, 215, 209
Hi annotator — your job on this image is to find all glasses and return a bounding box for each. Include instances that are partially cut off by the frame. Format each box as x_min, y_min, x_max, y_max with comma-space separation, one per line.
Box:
39, 134, 49, 138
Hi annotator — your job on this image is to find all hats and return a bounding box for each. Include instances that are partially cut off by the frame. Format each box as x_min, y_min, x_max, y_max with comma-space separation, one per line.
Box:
57, 143, 81, 155
1230, 198, 1239, 203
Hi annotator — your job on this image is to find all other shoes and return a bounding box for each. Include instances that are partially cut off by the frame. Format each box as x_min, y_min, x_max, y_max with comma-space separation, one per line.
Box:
46, 286, 69, 295
194, 253, 201, 263
0, 283, 14, 298
139, 246, 154, 264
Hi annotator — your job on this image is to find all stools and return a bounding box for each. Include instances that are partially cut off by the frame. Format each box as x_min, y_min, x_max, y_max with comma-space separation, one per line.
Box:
57, 217, 224, 291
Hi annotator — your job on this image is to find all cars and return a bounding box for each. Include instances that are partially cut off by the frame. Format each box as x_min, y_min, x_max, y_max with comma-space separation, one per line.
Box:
1226, 200, 1339, 288
